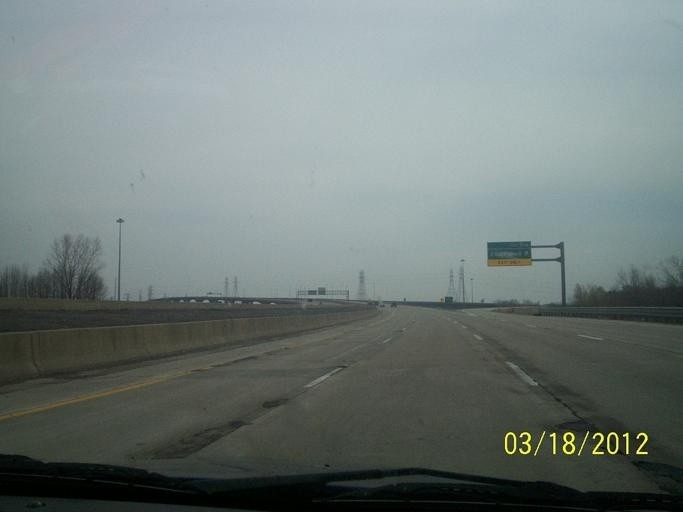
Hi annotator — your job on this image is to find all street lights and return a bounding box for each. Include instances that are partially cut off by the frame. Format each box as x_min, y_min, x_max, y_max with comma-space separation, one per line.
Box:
115, 217, 123, 301
